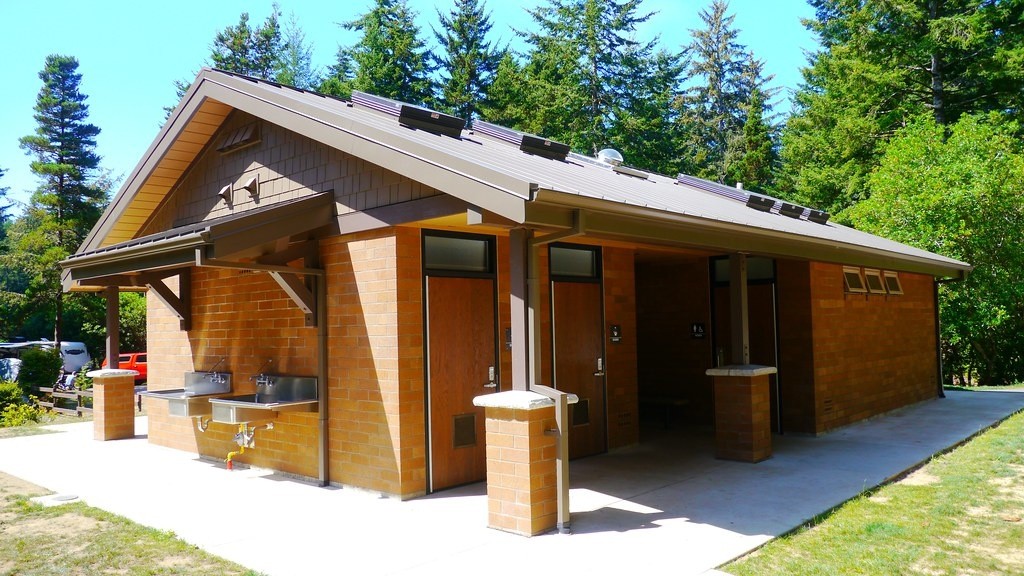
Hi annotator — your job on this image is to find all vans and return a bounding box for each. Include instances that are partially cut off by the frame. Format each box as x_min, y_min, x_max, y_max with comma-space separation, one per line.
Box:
0, 341, 91, 387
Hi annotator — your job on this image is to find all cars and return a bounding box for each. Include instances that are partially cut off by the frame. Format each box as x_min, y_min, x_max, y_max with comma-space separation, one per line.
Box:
102, 353, 147, 385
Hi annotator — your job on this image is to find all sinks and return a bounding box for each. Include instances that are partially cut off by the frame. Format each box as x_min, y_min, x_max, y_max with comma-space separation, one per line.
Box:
137, 371, 232, 418
208, 374, 318, 424
719, 365, 765, 370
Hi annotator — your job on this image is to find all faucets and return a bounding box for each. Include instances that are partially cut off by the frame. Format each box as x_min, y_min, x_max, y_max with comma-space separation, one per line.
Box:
249, 373, 275, 387
201, 371, 226, 383
716, 343, 724, 367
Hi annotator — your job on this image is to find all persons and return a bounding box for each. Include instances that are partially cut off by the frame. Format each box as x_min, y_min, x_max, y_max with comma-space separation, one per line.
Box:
64, 371, 77, 390
